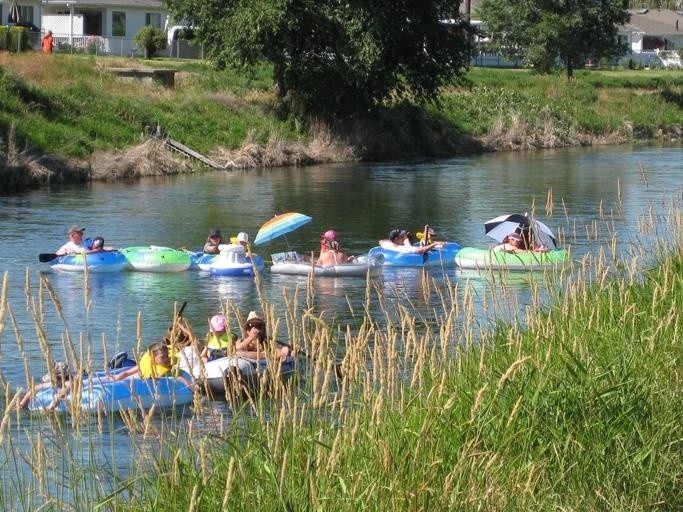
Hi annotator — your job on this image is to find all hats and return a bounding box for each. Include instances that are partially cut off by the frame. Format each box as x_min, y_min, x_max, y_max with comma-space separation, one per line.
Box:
207, 228, 222, 239
241, 310, 265, 328
235, 231, 249, 243
319, 229, 336, 240
68, 225, 86, 233
209, 314, 226, 332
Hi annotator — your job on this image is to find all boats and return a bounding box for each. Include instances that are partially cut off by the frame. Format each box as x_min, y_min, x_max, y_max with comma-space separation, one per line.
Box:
28, 358, 297, 417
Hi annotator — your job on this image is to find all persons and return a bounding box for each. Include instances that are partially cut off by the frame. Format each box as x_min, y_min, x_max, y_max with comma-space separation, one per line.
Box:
200, 314, 238, 363
16, 361, 81, 411
378, 229, 445, 254
235, 310, 286, 361
53, 225, 113, 255
319, 229, 335, 254
417, 225, 436, 246
40, 30, 53, 53
108, 342, 194, 392
493, 233, 547, 254
313, 238, 353, 266
230, 232, 253, 252
203, 229, 225, 254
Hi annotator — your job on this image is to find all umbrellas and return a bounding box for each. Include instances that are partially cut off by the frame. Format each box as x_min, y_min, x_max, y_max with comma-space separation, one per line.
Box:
253, 212, 312, 250
484, 212, 557, 250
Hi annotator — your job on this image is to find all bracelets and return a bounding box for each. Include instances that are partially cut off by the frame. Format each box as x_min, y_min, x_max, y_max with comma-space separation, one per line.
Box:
64, 248, 67, 255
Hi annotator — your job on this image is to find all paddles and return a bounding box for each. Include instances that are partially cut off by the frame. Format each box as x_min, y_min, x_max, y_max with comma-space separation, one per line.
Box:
262, 335, 357, 383
38, 250, 80, 263
423, 226, 429, 262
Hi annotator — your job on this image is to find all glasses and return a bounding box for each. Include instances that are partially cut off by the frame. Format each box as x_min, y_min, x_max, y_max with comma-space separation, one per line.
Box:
246, 322, 263, 331
